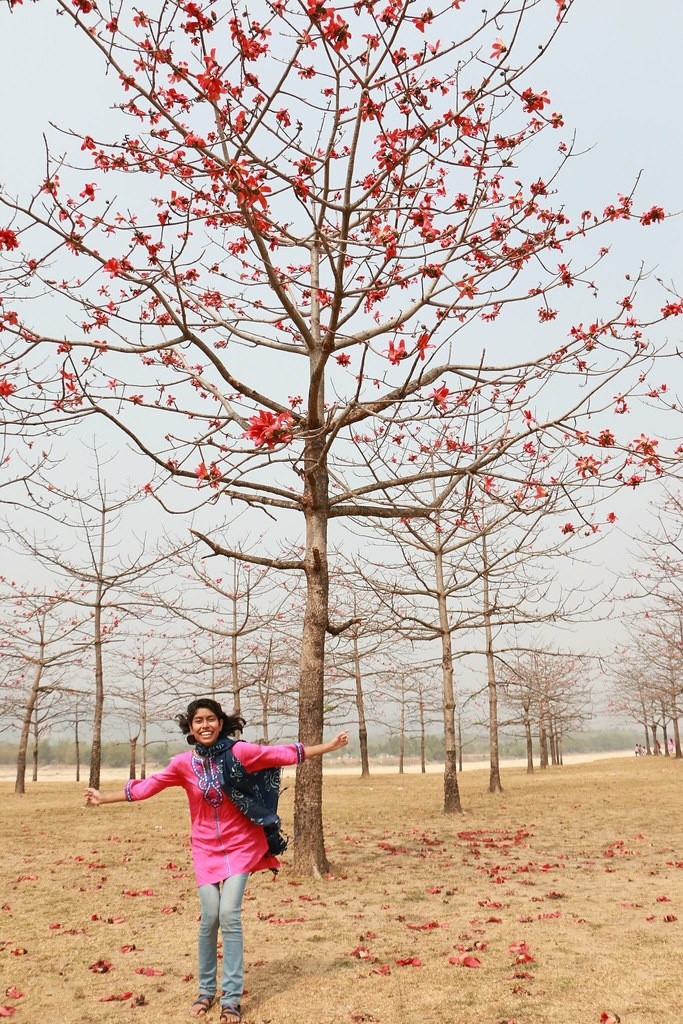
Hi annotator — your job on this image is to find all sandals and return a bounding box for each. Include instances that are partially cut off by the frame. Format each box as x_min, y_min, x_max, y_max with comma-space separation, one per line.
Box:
220, 1003, 241, 1024
188, 994, 217, 1017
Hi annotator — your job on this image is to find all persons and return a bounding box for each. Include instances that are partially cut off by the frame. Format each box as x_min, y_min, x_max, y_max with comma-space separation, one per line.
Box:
635, 738, 675, 756
84, 699, 351, 1024
